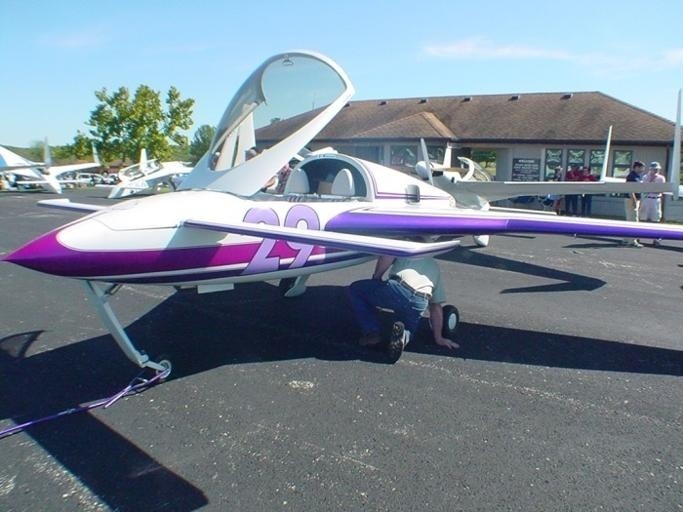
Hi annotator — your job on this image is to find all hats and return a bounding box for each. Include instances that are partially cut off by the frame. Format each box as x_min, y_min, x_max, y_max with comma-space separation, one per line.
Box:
649, 162, 661, 169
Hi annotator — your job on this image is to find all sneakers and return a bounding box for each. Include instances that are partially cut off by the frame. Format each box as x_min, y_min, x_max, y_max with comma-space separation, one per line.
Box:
633, 240, 644, 247
359, 321, 405, 363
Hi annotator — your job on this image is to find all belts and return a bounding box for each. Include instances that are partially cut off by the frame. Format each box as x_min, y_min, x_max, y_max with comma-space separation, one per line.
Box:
646, 196, 661, 198
390, 276, 432, 300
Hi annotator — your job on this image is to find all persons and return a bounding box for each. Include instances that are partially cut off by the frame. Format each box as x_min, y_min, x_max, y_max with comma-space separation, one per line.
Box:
552, 168, 565, 215
579, 166, 597, 218
638, 162, 666, 247
348, 254, 461, 364
564, 163, 583, 217
245, 147, 294, 196
621, 161, 647, 247
